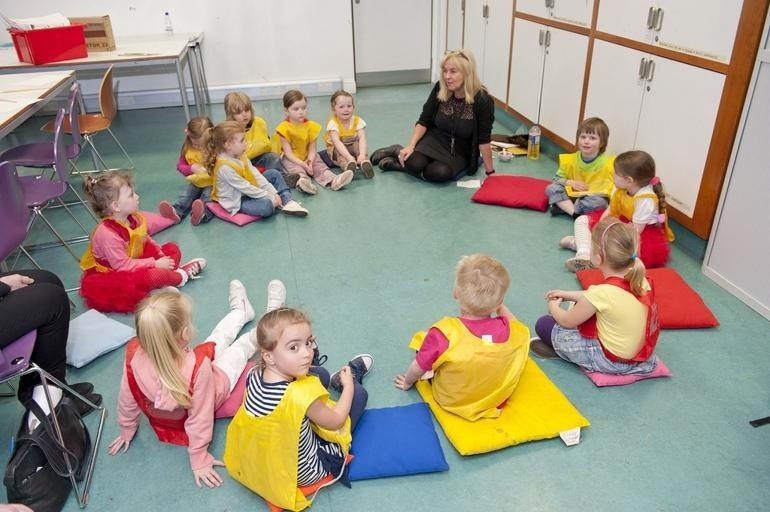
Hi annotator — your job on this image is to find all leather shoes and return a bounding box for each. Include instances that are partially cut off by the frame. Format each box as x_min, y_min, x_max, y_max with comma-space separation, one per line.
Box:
550, 203, 567, 215
73, 394, 103, 419
371, 145, 405, 167
379, 157, 403, 172
65, 382, 95, 400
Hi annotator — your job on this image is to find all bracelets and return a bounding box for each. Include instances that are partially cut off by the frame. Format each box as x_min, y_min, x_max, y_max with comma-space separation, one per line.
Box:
485, 169, 496, 175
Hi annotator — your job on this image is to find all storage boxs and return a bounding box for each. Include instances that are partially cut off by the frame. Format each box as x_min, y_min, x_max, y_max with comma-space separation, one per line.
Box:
5, 23, 88, 65
65, 14, 117, 53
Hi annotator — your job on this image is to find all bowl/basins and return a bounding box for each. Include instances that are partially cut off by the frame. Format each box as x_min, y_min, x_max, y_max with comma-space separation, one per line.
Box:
497, 151, 512, 162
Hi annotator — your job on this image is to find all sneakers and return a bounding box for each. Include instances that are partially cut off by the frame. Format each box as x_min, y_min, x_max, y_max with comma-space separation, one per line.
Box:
267, 280, 288, 315
330, 354, 375, 392
529, 337, 564, 362
559, 235, 578, 253
312, 339, 329, 367
180, 258, 208, 281
228, 280, 256, 324
160, 161, 376, 226
565, 257, 594, 274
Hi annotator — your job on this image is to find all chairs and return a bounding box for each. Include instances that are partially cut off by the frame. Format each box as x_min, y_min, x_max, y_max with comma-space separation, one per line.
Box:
0, 160, 78, 313
39, 63, 136, 175
0, 109, 100, 293
0, 87, 105, 249
0, 330, 107, 509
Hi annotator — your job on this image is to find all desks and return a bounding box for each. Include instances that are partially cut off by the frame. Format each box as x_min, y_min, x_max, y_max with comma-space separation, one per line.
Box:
0, 31, 211, 123
0, 71, 98, 170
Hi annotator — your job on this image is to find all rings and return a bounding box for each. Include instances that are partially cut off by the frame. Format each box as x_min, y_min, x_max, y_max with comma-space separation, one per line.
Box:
397, 155, 401, 159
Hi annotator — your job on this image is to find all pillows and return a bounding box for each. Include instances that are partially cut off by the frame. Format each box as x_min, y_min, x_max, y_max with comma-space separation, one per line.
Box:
576, 269, 719, 329
348, 403, 449, 481
136, 149, 336, 237
580, 355, 672, 388
264, 453, 354, 512
214, 360, 256, 419
414, 357, 590, 457
66, 307, 138, 368
470, 175, 553, 213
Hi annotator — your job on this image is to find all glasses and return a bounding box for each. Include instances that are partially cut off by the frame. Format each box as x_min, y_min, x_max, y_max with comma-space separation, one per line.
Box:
445, 50, 470, 63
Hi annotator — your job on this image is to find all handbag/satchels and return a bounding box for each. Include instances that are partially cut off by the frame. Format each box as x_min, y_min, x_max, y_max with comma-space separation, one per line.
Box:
4, 372, 92, 512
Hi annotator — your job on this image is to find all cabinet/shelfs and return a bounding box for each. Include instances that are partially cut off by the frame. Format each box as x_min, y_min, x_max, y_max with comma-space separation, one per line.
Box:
576, 32, 749, 241
462, 0, 515, 117
513, 0, 600, 36
591, 0, 769, 76
446, 1, 464, 53
506, 13, 591, 153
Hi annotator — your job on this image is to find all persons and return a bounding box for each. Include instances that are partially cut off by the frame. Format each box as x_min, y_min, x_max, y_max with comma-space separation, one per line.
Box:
223, 306, 374, 511
530, 215, 660, 376
394, 253, 530, 423
157, 91, 308, 227
78, 170, 207, 314
370, 48, 497, 186
0, 269, 104, 419
271, 89, 354, 196
108, 279, 287, 490
544, 116, 618, 219
560, 149, 676, 274
322, 90, 375, 181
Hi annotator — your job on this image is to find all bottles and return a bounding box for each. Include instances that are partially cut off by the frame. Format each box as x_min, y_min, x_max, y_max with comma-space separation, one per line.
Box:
527, 124, 541, 161
164, 13, 173, 38
